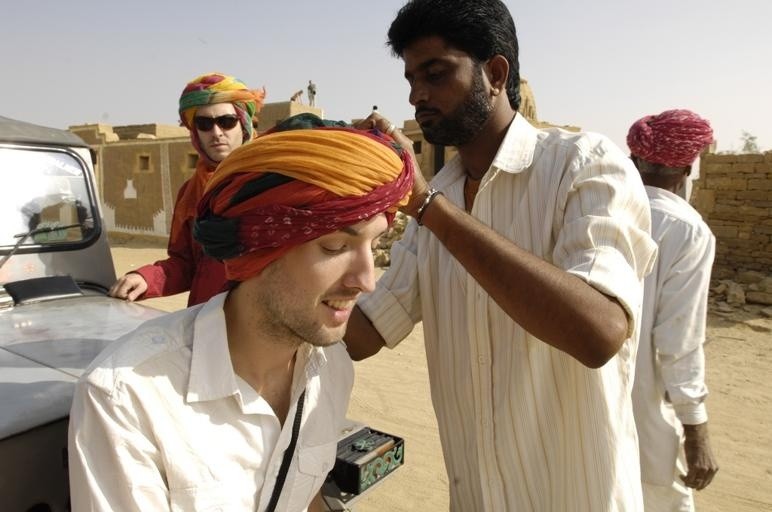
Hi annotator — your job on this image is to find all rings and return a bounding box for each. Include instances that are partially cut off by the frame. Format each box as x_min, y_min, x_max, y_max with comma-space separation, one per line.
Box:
385, 123, 396, 136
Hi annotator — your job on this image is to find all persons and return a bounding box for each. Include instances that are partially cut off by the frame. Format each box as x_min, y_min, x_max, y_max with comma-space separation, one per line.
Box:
624, 110, 721, 512
344, 0, 660, 512
291, 90, 303, 105
307, 79, 317, 106
99, 72, 271, 308
370, 105, 379, 115
59, 117, 417, 511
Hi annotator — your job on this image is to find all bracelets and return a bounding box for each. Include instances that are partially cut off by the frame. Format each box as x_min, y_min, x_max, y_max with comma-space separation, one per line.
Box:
412, 186, 446, 227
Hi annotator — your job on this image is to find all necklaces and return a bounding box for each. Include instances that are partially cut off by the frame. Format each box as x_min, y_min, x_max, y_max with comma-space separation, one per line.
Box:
464, 172, 487, 185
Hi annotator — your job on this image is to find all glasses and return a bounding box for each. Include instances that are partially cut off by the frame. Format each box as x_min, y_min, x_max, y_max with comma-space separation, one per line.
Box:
193, 115, 240, 131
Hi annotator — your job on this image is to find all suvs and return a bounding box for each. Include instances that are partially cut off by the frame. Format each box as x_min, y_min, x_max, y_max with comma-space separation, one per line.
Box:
0, 114, 412, 512
